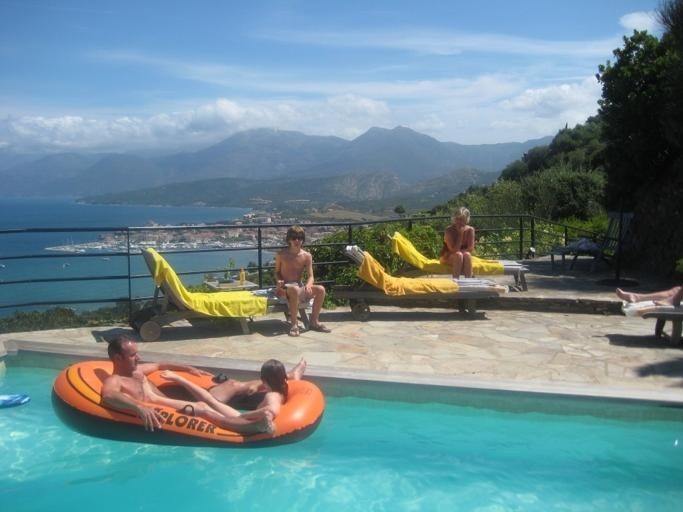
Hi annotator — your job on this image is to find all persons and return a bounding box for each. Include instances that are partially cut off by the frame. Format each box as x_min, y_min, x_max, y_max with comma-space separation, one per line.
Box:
271, 225, 332, 337
96, 336, 306, 434
613, 281, 682, 309
140, 359, 290, 420
439, 206, 475, 278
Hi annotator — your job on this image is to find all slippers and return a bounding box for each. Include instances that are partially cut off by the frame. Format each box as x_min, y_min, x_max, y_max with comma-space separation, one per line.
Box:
288, 322, 300, 336
310, 323, 331, 333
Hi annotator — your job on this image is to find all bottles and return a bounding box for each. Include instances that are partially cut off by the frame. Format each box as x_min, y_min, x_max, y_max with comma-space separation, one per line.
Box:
239, 268, 246, 286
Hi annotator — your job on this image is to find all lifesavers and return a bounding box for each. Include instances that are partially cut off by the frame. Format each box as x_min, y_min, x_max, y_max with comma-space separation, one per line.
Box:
0, 394, 30, 408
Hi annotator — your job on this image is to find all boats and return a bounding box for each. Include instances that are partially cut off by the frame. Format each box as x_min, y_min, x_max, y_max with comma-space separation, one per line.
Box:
53, 360, 325, 446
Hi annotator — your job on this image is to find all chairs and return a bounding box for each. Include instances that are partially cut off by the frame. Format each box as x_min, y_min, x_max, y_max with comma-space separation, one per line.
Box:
130, 247, 310, 341
545, 210, 635, 271
387, 232, 530, 292
331, 247, 509, 321
622, 301, 683, 347
